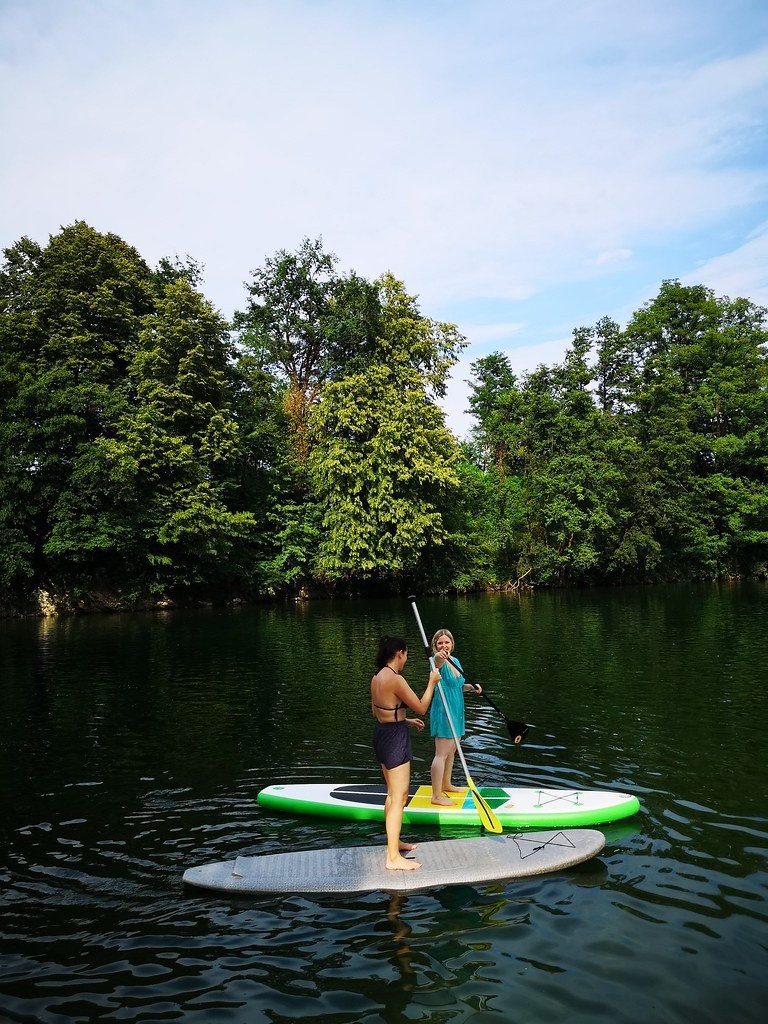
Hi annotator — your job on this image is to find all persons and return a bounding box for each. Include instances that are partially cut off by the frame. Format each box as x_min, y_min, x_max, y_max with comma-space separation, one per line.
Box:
429, 629, 481, 806
369, 635, 442, 870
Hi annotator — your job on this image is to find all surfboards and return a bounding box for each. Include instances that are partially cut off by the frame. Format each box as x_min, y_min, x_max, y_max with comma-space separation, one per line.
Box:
182, 829, 606, 893
256, 783, 641, 827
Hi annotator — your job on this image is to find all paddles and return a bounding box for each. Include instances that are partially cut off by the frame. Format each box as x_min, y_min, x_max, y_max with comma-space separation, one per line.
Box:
443, 650, 531, 746
408, 594, 503, 834
